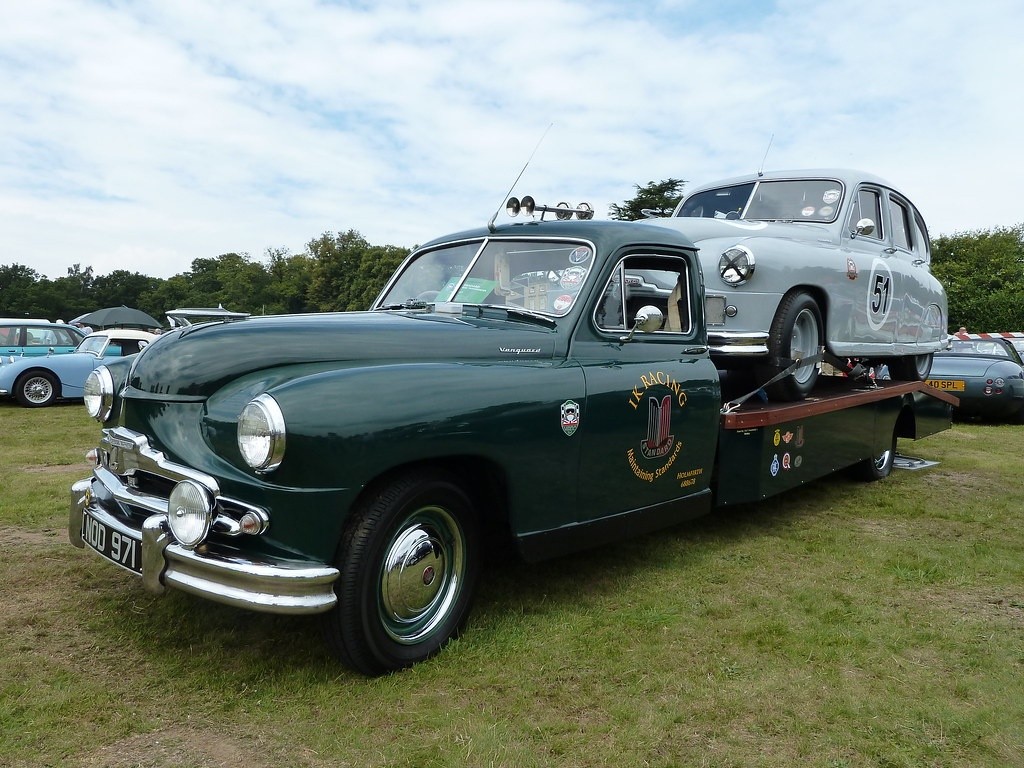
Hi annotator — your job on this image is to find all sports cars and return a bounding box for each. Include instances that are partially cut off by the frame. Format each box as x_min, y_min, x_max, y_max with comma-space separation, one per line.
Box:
876, 337, 1024, 420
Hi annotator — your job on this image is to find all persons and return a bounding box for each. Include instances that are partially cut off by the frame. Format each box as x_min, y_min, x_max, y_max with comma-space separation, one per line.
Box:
955, 327, 966, 335
76, 323, 93, 341
47, 319, 67, 344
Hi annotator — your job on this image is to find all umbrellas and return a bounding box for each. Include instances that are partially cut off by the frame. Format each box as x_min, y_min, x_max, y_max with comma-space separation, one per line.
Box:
77, 305, 164, 329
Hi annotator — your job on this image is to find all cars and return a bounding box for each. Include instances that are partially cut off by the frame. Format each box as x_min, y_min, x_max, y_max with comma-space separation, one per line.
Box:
633, 167, 953, 396
0, 330, 158, 408
0, 318, 122, 357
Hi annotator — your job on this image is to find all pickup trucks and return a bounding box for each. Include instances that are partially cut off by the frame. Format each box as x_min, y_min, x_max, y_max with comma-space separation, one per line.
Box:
68, 160, 952, 677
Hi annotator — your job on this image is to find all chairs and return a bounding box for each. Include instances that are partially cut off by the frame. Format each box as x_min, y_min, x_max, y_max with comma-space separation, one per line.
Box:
27, 332, 34, 345
